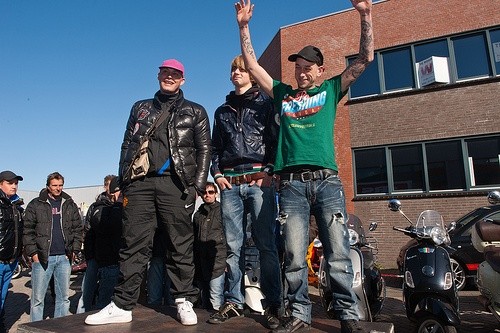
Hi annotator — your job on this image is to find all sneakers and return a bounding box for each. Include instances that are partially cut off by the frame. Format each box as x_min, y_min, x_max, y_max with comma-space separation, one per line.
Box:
340, 320, 368, 333
175, 298, 198, 325
270, 317, 311, 333
84, 301, 132, 324
208, 303, 244, 323
264, 306, 280, 329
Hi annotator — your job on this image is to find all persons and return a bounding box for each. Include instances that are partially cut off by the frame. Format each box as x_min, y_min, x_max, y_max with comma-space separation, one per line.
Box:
233, 0, 374, 333
0, 171, 228, 323
209, 53, 283, 328
85, 59, 211, 325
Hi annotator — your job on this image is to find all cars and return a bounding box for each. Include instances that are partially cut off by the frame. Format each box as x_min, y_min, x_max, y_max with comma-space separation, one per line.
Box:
397, 205, 500, 291
10, 246, 87, 279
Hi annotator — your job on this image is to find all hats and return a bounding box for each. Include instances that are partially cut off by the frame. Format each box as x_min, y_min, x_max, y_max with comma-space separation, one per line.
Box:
288, 45, 323, 66
0, 170, 23, 182
109, 177, 120, 193
158, 59, 184, 77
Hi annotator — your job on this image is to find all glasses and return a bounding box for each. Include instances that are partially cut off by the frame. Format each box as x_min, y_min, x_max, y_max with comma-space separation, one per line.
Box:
203, 191, 216, 195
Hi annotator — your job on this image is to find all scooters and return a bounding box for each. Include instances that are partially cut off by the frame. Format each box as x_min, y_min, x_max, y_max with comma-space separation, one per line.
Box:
388, 198, 463, 333
316, 213, 385, 322
470, 189, 500, 330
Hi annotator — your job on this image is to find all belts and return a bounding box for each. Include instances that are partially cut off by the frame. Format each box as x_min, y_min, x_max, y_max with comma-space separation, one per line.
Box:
224, 172, 267, 186
278, 168, 338, 183
